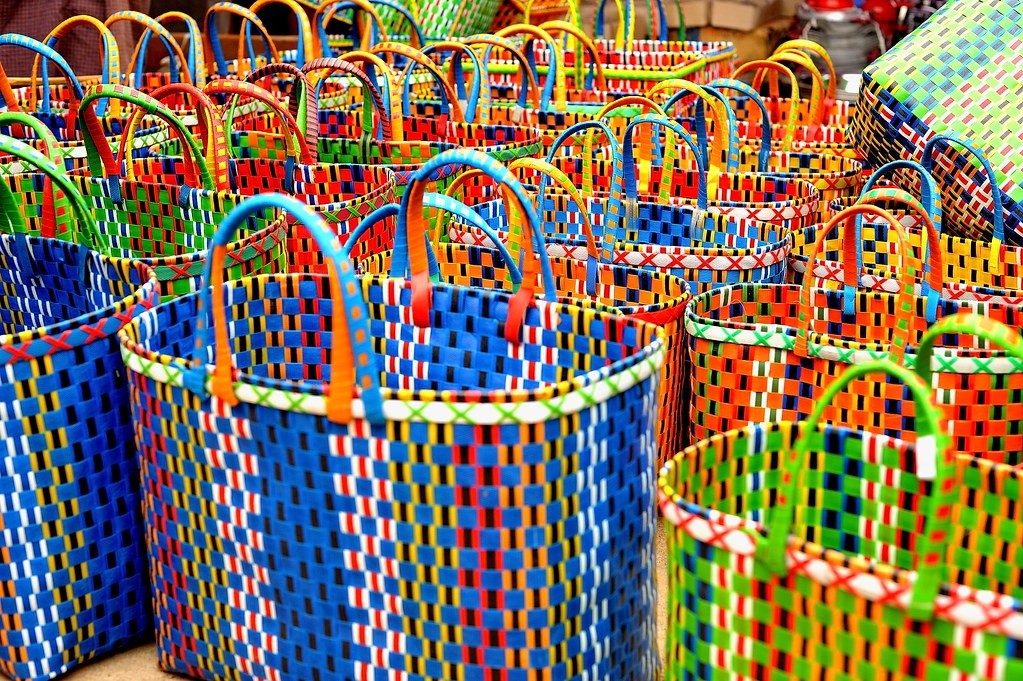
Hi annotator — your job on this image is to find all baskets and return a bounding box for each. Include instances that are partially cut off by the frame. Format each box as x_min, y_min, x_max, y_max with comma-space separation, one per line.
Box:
0, 1, 1023, 680
